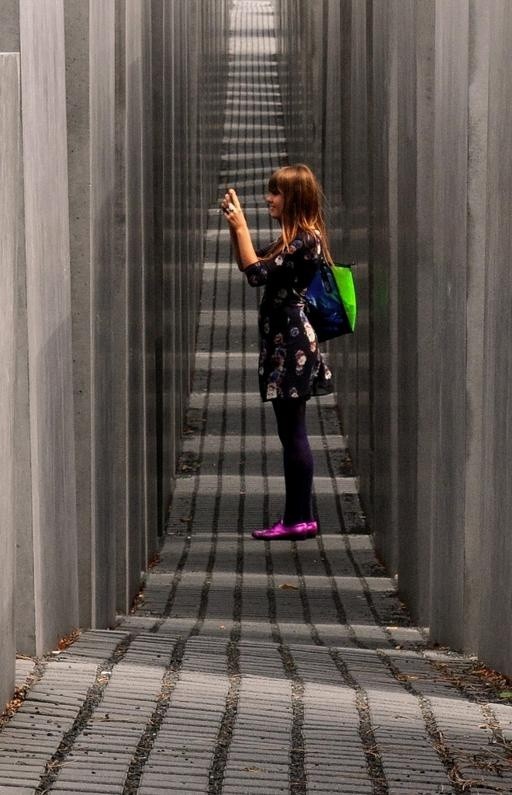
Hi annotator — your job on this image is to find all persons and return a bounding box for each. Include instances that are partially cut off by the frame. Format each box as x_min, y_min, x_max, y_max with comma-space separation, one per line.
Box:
220, 163, 335, 540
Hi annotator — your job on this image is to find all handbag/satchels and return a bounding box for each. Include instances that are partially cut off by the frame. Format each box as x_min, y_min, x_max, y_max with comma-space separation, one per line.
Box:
305, 263, 358, 343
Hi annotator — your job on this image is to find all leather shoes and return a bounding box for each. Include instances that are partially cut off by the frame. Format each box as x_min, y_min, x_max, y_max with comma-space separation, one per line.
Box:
252, 513, 318, 540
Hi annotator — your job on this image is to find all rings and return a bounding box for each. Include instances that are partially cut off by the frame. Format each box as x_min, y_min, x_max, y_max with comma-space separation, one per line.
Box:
227, 203, 235, 212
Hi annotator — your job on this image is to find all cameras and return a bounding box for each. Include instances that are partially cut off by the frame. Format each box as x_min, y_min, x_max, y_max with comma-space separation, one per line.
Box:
223, 203, 236, 217
